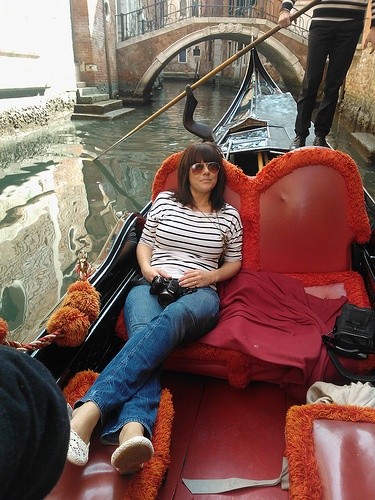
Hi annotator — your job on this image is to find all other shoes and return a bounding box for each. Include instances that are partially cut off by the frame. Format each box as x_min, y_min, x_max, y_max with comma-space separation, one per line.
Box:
61, 414, 93, 468
109, 433, 155, 477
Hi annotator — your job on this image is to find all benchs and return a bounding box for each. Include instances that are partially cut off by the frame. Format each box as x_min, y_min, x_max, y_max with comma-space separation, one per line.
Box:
118, 146, 370, 388
285, 402, 375, 500
42, 369, 174, 499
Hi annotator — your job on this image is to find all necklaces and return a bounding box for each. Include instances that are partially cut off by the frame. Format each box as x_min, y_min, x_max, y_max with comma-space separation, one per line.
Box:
197, 206, 229, 251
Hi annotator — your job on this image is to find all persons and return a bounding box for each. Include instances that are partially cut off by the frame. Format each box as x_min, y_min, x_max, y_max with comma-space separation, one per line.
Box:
0, 345, 71, 500
278, 0, 375, 151
66, 141, 244, 476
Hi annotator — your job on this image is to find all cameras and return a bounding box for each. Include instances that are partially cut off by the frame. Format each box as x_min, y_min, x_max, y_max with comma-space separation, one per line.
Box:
149, 276, 192, 306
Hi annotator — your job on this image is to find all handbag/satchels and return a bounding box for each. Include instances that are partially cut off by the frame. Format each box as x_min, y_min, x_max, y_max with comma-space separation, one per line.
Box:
321, 306, 375, 383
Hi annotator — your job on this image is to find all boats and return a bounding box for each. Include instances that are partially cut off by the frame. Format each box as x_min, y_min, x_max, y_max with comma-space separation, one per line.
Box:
27, 41, 375, 500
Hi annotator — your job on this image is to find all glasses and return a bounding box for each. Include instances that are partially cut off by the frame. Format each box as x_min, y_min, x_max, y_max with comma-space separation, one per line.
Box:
190, 161, 221, 175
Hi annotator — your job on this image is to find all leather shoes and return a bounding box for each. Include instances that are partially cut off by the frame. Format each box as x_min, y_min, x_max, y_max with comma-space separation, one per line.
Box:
289, 134, 306, 151
312, 135, 330, 149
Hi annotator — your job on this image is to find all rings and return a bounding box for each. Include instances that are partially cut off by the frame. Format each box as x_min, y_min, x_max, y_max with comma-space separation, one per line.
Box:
195, 281, 197, 284
